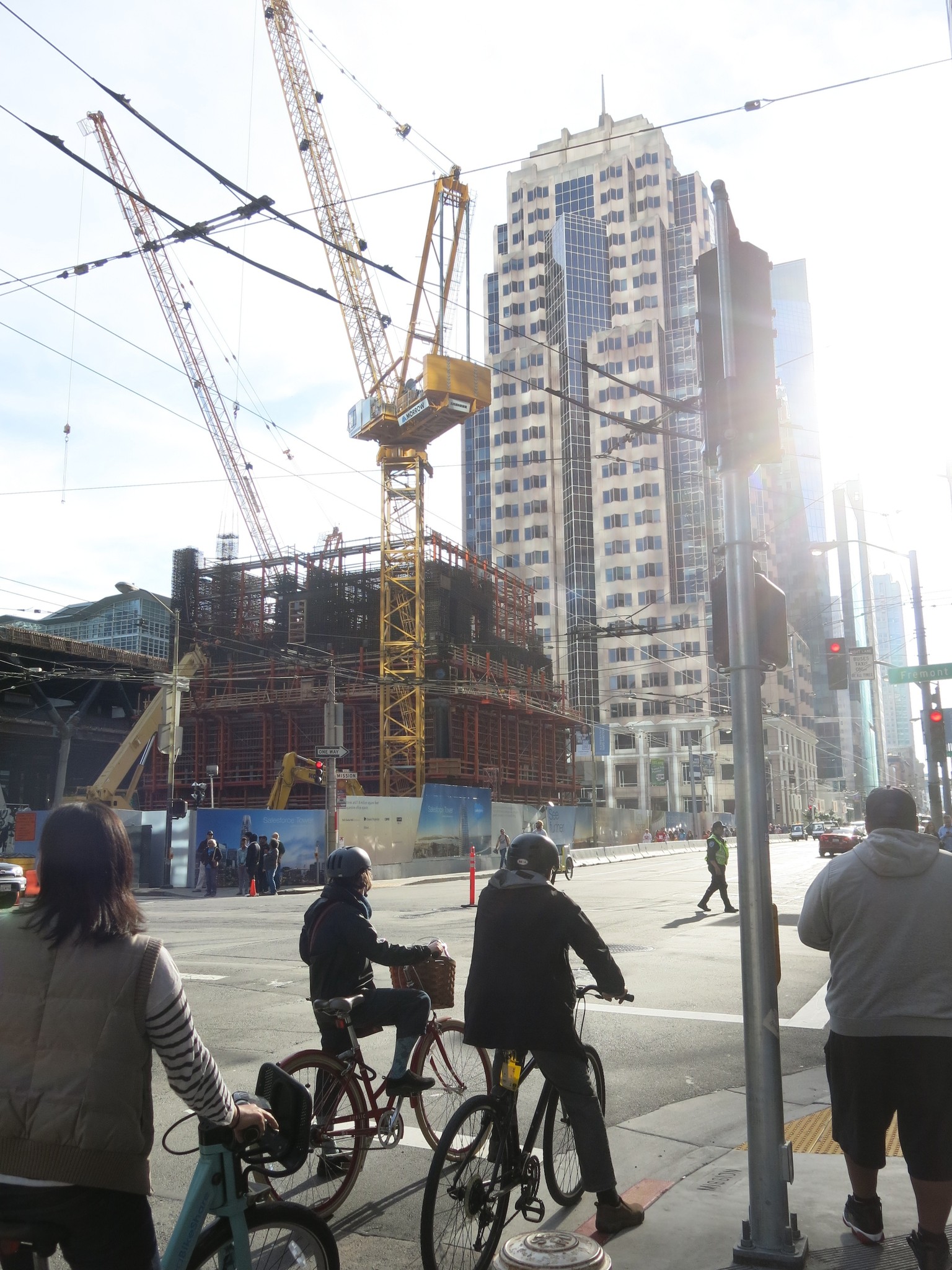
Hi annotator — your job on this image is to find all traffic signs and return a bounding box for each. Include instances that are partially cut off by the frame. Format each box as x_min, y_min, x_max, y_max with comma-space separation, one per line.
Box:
315, 745, 350, 759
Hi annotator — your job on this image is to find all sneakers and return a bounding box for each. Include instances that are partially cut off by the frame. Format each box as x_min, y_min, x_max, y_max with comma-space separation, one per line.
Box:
842, 1193, 886, 1246
911, 1226, 951, 1270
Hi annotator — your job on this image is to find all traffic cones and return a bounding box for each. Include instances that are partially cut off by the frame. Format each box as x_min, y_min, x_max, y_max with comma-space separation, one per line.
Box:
245, 879, 259, 897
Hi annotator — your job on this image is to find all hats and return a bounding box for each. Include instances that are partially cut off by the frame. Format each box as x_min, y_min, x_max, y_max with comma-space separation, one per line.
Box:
866, 785, 916, 820
713, 822, 726, 828
259, 836, 267, 841
207, 831, 213, 835
501, 829, 505, 832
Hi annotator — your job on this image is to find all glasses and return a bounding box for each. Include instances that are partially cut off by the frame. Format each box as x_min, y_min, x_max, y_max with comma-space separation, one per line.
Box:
208, 843, 213, 844
536, 822, 542, 826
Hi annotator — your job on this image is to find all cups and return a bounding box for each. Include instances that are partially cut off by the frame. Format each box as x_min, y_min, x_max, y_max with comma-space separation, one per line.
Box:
264, 849, 268, 853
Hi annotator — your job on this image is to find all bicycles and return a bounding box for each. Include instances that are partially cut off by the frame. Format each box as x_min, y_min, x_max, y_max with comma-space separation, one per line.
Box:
251, 935, 493, 1222
420, 985, 633, 1269
160, 1091, 341, 1270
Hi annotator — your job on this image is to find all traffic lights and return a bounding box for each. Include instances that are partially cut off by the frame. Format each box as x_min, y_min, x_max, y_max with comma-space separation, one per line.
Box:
809, 805, 812, 812
775, 803, 780, 812
314, 760, 323, 784
928, 709, 945, 743
825, 637, 848, 690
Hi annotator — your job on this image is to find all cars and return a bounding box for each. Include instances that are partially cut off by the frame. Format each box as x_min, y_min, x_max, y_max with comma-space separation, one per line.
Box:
0, 862, 27, 910
789, 823, 808, 842
811, 820, 869, 857
916, 812, 931, 832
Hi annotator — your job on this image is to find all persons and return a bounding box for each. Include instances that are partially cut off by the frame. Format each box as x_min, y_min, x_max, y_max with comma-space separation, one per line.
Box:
696, 820, 739, 913
492, 828, 511, 870
462, 834, 644, 1235
296, 845, 444, 1174
0, 799, 279, 1270
191, 828, 285, 898
532, 819, 548, 839
797, 785, 952, 1270
642, 822, 793, 846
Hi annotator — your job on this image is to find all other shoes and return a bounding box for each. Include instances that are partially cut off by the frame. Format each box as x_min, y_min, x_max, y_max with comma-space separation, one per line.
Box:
487, 1139, 514, 1164
236, 887, 281, 895
382, 1070, 435, 1096
315, 1149, 364, 1175
211, 894, 215, 897
725, 907, 739, 913
204, 893, 210, 896
500, 867, 502, 869
594, 1197, 645, 1233
698, 902, 711, 911
192, 889, 201, 892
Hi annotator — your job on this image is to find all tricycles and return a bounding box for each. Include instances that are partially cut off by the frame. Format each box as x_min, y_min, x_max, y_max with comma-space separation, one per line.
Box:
544, 843, 574, 886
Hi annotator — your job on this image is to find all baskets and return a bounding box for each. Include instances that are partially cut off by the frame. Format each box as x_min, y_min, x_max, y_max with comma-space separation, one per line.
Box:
390, 955, 456, 1010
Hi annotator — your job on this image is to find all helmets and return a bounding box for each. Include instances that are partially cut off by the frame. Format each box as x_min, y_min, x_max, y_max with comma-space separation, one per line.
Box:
325, 845, 371, 878
505, 834, 560, 877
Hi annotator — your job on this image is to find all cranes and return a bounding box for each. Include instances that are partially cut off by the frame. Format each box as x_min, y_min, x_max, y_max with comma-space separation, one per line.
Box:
85, 110, 286, 581
262, 0, 492, 797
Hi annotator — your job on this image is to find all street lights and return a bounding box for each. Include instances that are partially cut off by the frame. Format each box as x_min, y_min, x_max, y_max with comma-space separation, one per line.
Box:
809, 539, 945, 830
114, 583, 181, 889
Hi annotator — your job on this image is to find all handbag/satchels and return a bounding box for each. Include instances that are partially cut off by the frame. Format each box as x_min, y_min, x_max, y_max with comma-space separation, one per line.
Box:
213, 847, 218, 867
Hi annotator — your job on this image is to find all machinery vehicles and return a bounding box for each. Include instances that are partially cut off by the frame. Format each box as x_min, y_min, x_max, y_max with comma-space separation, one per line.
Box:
266, 752, 364, 810
